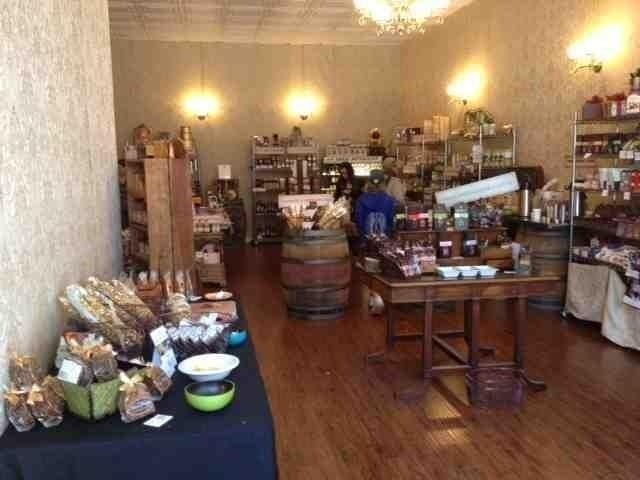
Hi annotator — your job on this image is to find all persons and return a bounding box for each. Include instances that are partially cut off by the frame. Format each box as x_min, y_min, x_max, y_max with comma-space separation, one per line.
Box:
332, 157, 429, 272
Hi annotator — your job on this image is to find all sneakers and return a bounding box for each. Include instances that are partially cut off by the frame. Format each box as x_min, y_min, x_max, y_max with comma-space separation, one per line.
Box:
368, 296, 384, 315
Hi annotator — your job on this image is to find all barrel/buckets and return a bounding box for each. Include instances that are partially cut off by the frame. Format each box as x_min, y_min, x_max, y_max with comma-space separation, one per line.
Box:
280, 230, 351, 320
515, 231, 569, 311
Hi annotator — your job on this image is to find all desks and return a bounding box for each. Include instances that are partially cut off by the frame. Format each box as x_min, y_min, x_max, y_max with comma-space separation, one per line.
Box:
194, 227, 229, 287
0, 299, 279, 479
354, 256, 565, 387
394, 226, 508, 252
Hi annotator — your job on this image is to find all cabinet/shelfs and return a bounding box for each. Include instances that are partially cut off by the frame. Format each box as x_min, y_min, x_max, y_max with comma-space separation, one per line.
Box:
123, 156, 197, 295
559, 110, 639, 355
323, 155, 382, 195
391, 133, 446, 206
446, 127, 515, 196
250, 141, 320, 243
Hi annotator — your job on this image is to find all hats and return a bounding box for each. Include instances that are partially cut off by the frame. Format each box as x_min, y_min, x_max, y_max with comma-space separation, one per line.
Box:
370, 169, 384, 184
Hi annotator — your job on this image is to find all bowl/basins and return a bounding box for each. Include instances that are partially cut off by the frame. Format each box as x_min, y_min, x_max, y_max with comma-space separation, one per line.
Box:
178, 353, 240, 381
185, 379, 235, 412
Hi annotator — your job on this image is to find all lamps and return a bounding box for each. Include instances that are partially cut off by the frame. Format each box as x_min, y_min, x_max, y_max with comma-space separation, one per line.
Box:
353, 0, 452, 39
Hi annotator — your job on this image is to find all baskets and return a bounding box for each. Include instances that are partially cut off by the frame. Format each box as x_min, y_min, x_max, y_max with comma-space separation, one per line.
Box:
68, 304, 234, 360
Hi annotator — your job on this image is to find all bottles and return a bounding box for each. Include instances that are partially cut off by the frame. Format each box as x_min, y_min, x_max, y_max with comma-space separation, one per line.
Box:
453, 149, 513, 172
